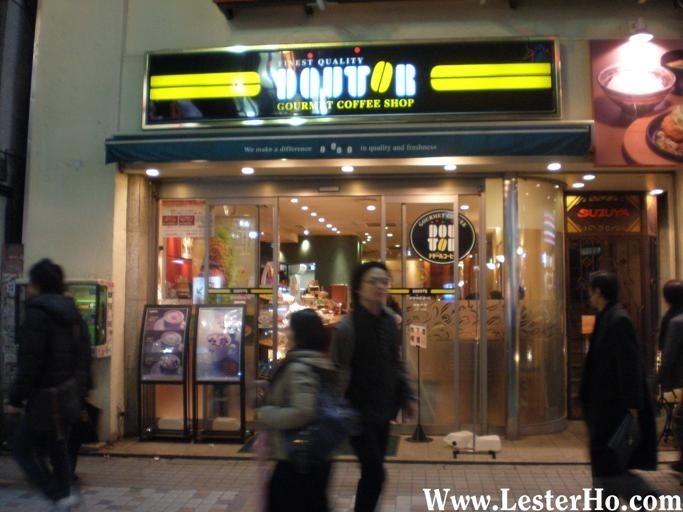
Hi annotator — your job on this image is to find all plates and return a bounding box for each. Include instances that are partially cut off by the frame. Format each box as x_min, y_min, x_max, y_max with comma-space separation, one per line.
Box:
645, 109, 683, 161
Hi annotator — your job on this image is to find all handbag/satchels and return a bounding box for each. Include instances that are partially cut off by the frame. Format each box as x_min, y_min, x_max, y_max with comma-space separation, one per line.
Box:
29, 385, 82, 435
605, 413, 642, 475
288, 395, 352, 459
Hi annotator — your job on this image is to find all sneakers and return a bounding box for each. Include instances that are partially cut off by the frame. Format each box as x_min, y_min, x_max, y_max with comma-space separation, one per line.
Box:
53, 494, 81, 511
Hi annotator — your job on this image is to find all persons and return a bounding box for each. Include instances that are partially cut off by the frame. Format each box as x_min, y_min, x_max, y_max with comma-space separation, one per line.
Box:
5, 256, 94, 512
25, 281, 85, 479
576, 268, 665, 512
249, 306, 339, 510
655, 278, 681, 473
326, 260, 418, 512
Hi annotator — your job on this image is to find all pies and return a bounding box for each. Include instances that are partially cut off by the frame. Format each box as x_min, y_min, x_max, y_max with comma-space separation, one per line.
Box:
655, 113, 682, 156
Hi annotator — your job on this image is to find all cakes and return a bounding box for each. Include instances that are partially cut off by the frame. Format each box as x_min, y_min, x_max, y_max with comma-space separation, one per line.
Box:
160, 332, 181, 352
160, 354, 179, 375
163, 311, 184, 330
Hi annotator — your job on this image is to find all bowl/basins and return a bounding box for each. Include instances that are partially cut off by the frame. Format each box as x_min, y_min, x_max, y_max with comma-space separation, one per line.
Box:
596, 63, 677, 119
